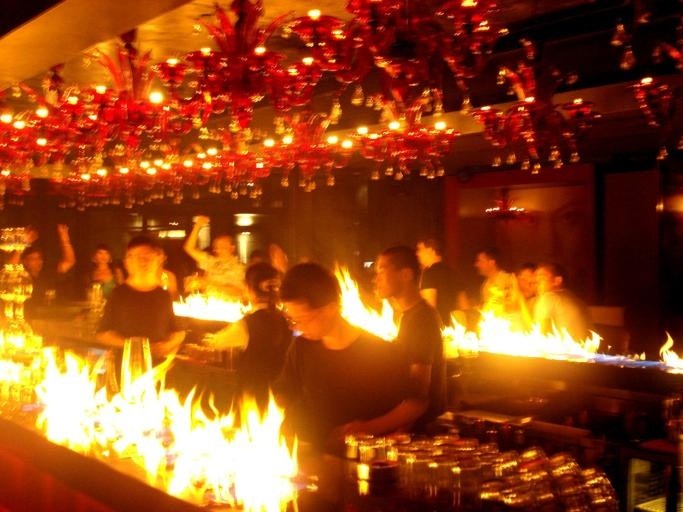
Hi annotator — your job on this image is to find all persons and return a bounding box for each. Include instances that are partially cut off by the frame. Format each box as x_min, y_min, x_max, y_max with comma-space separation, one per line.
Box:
0, 211, 470, 444
476, 246, 593, 343
